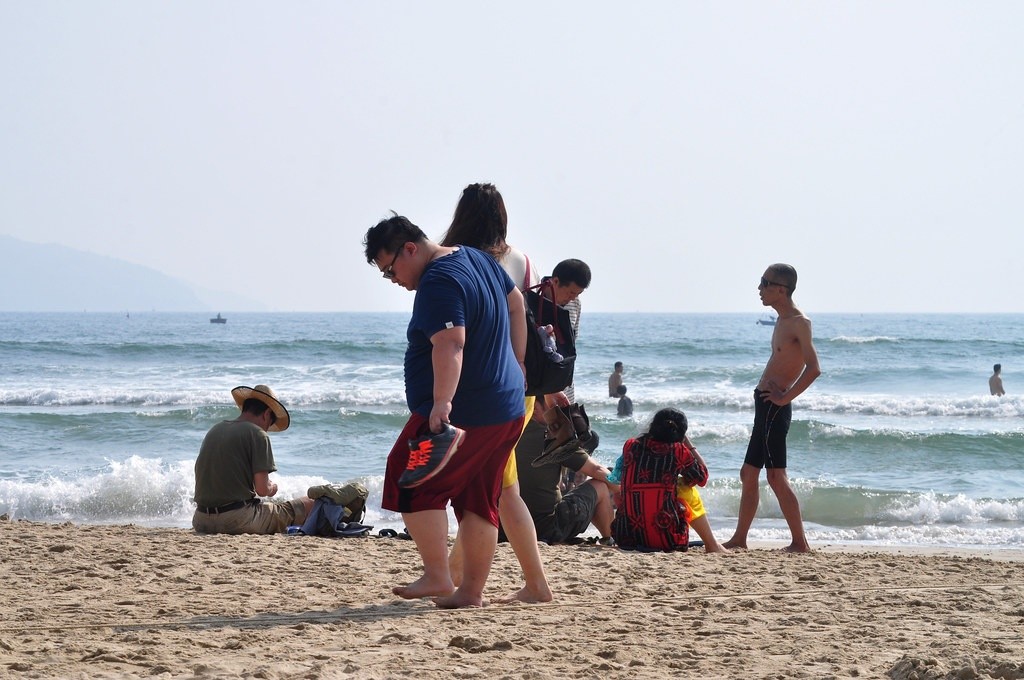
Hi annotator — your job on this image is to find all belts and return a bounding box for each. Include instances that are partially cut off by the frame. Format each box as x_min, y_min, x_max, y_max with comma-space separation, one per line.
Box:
198, 498, 253, 514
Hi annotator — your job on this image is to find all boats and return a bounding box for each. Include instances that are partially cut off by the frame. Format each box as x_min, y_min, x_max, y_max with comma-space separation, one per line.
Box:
755, 315, 776, 326
210, 313, 227, 323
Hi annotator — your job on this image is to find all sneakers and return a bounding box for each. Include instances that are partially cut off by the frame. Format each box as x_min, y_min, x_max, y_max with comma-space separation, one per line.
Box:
397, 422, 466, 489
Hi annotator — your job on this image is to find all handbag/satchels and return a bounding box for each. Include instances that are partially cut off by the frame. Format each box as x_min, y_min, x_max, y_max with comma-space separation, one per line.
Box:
521, 279, 577, 397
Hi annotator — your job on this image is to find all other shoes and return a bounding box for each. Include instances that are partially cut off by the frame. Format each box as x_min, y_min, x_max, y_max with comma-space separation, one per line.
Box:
530, 403, 599, 468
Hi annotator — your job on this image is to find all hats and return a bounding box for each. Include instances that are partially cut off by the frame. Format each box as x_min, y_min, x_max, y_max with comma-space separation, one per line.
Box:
231, 385, 290, 432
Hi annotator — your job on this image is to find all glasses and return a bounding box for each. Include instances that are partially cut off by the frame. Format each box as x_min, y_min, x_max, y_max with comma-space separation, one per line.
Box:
761, 277, 790, 289
383, 243, 405, 278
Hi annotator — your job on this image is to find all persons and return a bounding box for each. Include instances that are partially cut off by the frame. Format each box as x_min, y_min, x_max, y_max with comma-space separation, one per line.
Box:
617, 385, 633, 417
518, 259, 618, 541
609, 362, 623, 398
194, 384, 315, 533
989, 363, 1005, 396
364, 182, 553, 609
727, 265, 822, 555
611, 408, 733, 555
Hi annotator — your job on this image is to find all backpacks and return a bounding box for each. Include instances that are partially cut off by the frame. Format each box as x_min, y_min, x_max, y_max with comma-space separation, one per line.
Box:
298, 495, 374, 538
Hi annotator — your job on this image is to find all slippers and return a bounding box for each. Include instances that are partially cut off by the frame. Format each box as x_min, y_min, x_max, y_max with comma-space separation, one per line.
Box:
375, 528, 412, 540
